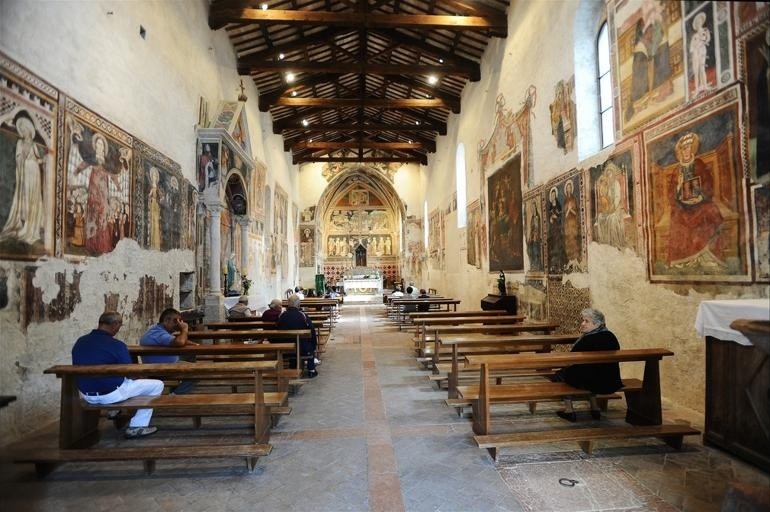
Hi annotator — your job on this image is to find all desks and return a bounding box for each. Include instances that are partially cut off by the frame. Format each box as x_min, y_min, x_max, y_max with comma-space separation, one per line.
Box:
466, 348, 675, 435
280, 297, 343, 321
125, 343, 295, 393
43, 359, 278, 444
413, 316, 525, 367
411, 335, 524, 351
413, 309, 508, 349
383, 292, 463, 309
425, 322, 560, 371
174, 329, 311, 374
442, 333, 580, 402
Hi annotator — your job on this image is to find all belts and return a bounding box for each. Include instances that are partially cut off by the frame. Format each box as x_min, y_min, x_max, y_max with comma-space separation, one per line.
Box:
76, 379, 124, 396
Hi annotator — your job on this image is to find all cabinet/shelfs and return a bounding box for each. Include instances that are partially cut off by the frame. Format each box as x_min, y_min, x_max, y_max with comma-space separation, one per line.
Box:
701, 298, 770, 479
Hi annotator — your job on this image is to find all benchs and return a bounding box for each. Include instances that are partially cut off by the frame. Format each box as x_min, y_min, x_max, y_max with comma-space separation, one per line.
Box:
432, 363, 566, 381
445, 394, 622, 416
173, 368, 303, 393
419, 347, 543, 367
193, 354, 312, 366
2, 445, 274, 475
89, 406, 293, 428
456, 378, 642, 420
473, 424, 701, 463
386, 309, 412, 328
427, 369, 559, 389
110, 392, 289, 426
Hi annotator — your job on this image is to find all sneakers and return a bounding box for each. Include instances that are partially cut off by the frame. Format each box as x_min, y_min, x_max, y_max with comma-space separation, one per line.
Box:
305, 357, 322, 366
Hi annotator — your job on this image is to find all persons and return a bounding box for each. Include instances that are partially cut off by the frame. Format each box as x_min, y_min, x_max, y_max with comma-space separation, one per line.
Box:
405, 281, 419, 294
339, 238, 348, 255
228, 294, 263, 343
72, 311, 164, 438
379, 238, 385, 255
392, 286, 404, 296
277, 295, 319, 379
416, 289, 430, 312
385, 237, 392, 255
0, 117, 52, 246
140, 308, 200, 395
74, 132, 121, 254
371, 238, 377, 256
163, 173, 182, 248
146, 166, 170, 252
293, 287, 304, 300
326, 286, 340, 297
558, 180, 578, 264
690, 11, 711, 99
667, 132, 723, 272
262, 299, 282, 339
335, 238, 339, 254
527, 201, 541, 268
544, 309, 625, 422
597, 162, 624, 246
328, 237, 335, 255
227, 253, 242, 291
323, 288, 334, 298
631, 0, 671, 105
492, 186, 517, 264
546, 187, 564, 264
401, 287, 416, 311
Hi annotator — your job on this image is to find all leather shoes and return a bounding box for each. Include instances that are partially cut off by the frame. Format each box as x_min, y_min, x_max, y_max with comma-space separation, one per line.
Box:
590, 410, 601, 421
126, 427, 157, 438
308, 370, 319, 378
557, 410, 576, 423
107, 409, 120, 419
